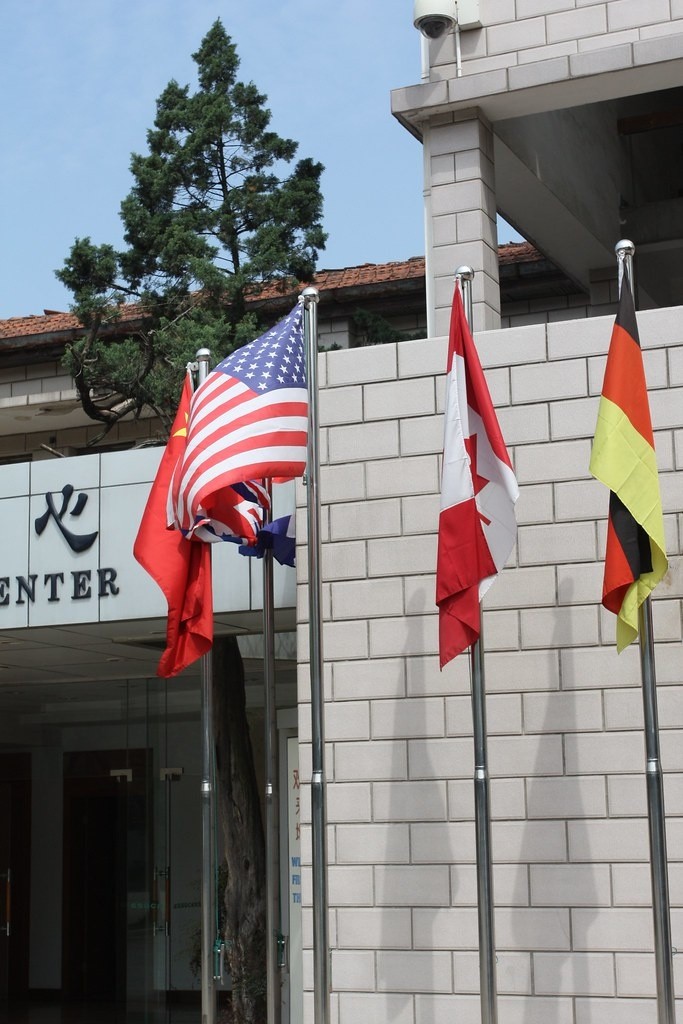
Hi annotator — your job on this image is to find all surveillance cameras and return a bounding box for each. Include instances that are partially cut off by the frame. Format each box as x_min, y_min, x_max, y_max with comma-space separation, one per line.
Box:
413, 0, 457, 41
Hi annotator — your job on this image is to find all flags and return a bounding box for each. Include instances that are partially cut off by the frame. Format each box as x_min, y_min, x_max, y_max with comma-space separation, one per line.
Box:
436, 280, 519, 672
132, 367, 213, 677
165, 300, 308, 568
589, 261, 668, 654
